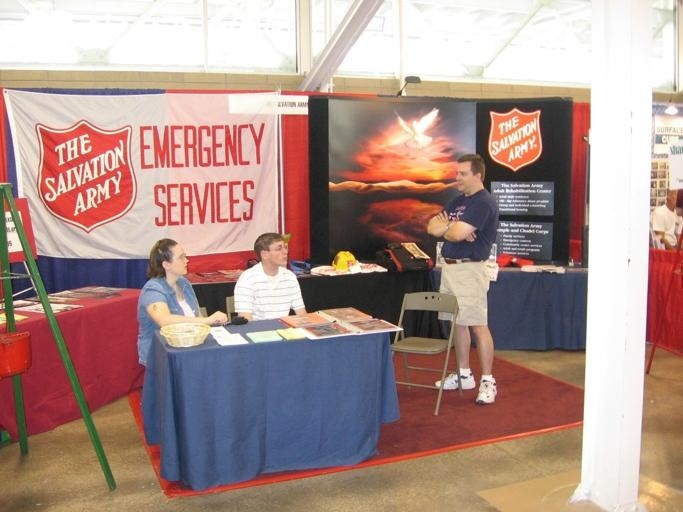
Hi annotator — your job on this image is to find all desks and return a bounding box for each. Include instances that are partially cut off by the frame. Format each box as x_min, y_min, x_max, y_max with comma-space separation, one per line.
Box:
487, 267, 588, 350
188, 264, 451, 343
152, 305, 391, 493
0, 283, 145, 447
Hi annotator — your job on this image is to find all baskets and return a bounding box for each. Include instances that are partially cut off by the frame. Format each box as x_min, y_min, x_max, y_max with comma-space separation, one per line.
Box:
159, 324, 211, 347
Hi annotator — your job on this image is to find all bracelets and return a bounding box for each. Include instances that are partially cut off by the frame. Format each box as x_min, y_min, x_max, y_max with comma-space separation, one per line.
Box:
446, 221, 451, 230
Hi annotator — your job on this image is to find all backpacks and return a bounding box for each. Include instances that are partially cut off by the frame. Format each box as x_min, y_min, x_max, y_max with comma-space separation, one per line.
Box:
375, 241, 434, 273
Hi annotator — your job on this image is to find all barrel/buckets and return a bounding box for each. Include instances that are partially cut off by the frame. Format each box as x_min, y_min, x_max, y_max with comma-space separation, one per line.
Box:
0, 319, 32, 379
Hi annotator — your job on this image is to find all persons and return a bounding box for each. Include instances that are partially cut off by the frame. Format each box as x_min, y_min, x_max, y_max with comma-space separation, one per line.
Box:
427, 155, 499, 404
234, 233, 307, 321
137, 238, 227, 366
653, 190, 682, 249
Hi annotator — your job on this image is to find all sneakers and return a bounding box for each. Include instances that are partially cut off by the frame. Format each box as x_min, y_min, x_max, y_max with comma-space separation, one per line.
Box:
476, 381, 498, 404
435, 374, 477, 390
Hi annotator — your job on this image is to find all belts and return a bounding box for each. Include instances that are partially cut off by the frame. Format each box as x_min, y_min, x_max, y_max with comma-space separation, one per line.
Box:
444, 256, 470, 265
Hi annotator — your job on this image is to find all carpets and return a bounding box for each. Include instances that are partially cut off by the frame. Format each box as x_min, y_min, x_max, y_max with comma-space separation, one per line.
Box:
126, 337, 586, 499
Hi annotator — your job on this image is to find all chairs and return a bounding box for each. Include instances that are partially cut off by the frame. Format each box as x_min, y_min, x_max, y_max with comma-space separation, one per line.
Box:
386, 292, 464, 417
